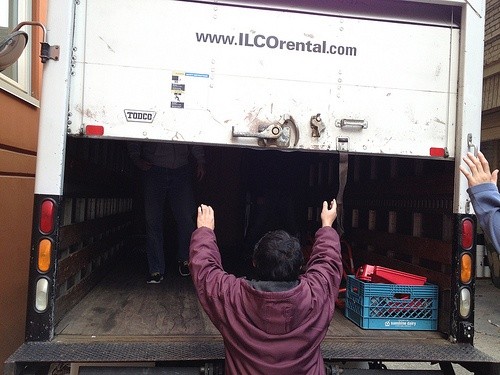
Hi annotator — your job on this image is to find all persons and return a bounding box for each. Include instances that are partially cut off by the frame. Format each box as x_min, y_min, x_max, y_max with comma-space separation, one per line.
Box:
458, 151, 500, 257
190, 199, 342, 375
126, 140, 206, 285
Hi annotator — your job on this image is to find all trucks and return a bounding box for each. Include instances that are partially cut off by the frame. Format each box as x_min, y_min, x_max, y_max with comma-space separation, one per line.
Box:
0, 0, 500, 375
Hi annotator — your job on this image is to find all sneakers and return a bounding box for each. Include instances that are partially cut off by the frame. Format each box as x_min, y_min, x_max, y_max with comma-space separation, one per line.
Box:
146, 273, 164, 284
179, 262, 190, 276
